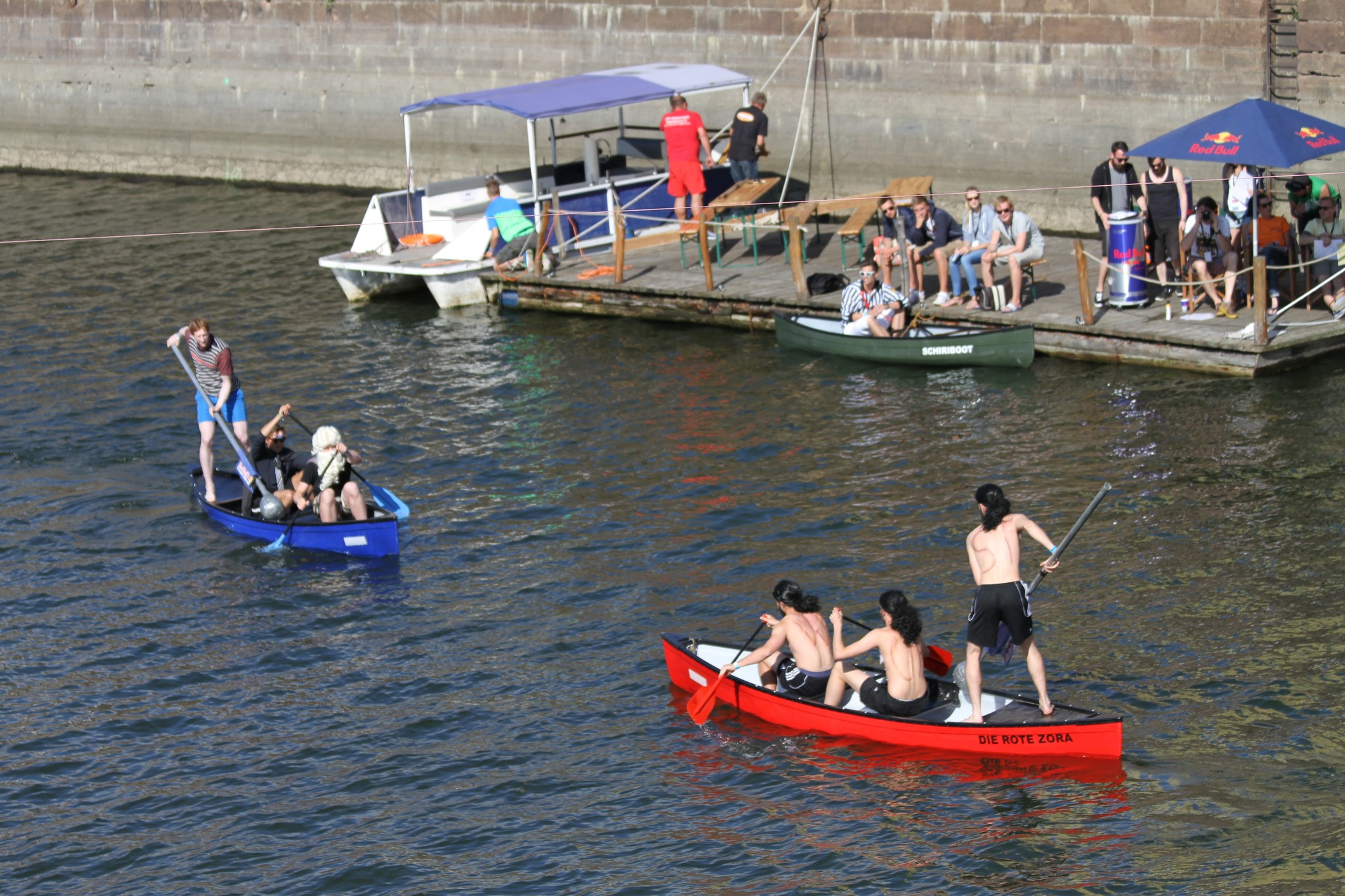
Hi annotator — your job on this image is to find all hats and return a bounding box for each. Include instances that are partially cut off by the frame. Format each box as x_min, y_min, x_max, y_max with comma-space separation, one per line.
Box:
1285, 172, 1310, 192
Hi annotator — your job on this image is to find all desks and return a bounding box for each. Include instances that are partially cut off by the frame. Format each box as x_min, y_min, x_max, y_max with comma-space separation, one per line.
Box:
709, 177, 781, 266
883, 176, 932, 206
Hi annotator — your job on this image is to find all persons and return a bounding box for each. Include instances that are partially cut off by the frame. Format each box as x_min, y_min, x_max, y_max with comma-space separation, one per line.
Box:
293, 424, 368, 524
484, 178, 555, 273
839, 259, 911, 339
659, 95, 718, 241
1089, 140, 1345, 320
981, 195, 1045, 313
717, 580, 836, 698
940, 185, 996, 309
166, 317, 249, 503
250, 403, 319, 520
910, 195, 963, 307
957, 484, 1062, 724
728, 92, 770, 218
876, 195, 919, 305
822, 588, 939, 718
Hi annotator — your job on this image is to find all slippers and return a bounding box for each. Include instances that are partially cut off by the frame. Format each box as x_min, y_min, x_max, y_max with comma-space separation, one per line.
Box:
1001, 303, 1023, 313
1267, 305, 1278, 316
973, 302, 981, 309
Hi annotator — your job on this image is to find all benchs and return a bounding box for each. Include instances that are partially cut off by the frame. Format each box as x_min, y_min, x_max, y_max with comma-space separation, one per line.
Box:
677, 204, 726, 267
781, 203, 819, 264
838, 204, 880, 271
926, 249, 1046, 301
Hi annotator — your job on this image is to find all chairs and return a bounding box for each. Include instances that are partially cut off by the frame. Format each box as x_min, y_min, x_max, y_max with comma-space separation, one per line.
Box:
1242, 223, 1296, 308
1177, 228, 1251, 313
1305, 244, 1323, 310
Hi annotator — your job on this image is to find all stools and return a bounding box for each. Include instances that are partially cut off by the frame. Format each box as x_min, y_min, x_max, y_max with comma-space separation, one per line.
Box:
860, 700, 953, 720
781, 682, 850, 700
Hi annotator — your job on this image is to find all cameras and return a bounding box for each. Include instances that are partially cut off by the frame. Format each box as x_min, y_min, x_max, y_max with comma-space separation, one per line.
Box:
1201, 210, 1211, 221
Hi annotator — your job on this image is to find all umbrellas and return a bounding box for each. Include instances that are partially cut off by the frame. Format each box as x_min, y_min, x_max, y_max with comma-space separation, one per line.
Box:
1122, 95, 1345, 258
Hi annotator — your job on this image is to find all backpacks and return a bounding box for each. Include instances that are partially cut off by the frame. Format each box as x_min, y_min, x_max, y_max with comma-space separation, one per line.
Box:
807, 272, 850, 295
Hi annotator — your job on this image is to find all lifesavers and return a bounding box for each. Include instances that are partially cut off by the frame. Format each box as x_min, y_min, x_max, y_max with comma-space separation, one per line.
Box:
400, 233, 444, 246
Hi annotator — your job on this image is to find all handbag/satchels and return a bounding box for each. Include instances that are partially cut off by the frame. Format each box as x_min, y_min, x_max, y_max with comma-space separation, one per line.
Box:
873, 236, 900, 267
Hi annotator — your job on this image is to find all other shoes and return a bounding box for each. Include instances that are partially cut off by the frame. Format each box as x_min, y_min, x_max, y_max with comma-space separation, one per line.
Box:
524, 248, 535, 273
1332, 295, 1345, 319
541, 252, 553, 272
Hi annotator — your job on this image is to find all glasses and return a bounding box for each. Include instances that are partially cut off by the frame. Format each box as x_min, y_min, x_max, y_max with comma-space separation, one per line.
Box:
996, 207, 1011, 214
882, 206, 894, 213
1259, 201, 1273, 207
967, 196, 979, 201
1115, 156, 1129, 163
859, 271, 875, 277
1318, 205, 1334, 210
1150, 162, 1163, 169
269, 435, 285, 443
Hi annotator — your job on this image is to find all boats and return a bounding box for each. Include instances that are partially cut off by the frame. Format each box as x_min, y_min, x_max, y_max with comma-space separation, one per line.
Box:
318, 61, 812, 312
771, 309, 1036, 367
185, 461, 400, 558
659, 630, 1123, 759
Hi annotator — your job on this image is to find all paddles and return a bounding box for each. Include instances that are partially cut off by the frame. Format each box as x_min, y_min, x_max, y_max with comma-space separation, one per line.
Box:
282, 411, 410, 520
836, 605, 953, 678
261, 450, 338, 552
686, 614, 767, 725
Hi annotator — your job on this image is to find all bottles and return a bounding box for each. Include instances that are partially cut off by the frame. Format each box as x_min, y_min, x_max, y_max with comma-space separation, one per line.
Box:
1165, 300, 1172, 320
1240, 291, 1244, 304
1192, 295, 1208, 302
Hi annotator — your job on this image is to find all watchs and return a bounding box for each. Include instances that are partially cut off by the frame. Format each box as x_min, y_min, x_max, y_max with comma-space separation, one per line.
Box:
1214, 228, 1222, 235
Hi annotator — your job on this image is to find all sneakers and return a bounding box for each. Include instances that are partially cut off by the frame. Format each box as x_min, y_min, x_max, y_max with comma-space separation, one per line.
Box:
903, 290, 925, 303
1094, 290, 1104, 308
1214, 297, 1237, 318
1154, 285, 1172, 300
697, 230, 716, 240
1175, 275, 1183, 296
933, 291, 950, 305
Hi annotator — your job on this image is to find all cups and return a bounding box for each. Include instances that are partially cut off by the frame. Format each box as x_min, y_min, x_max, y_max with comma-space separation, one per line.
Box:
1180, 298, 1189, 312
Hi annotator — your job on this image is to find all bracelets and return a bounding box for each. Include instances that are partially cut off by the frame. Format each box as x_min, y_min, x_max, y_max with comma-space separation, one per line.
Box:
1295, 208, 1302, 215
342, 450, 348, 455
175, 333, 181, 340
733, 662, 740, 670
1049, 545, 1058, 554
277, 413, 283, 420
488, 247, 496, 252
